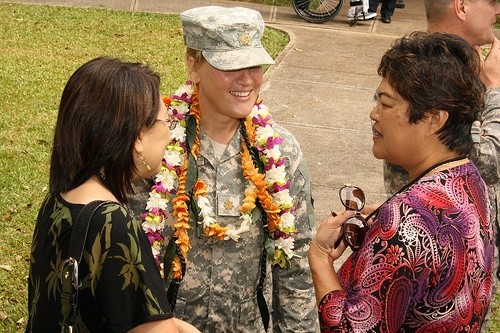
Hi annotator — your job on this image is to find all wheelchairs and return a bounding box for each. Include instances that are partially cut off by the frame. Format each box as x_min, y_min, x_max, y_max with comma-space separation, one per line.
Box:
293, 0, 375, 27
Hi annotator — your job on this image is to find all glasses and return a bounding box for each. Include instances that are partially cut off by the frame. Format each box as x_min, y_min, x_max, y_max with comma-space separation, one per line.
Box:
156, 117, 180, 130
331, 184, 368, 251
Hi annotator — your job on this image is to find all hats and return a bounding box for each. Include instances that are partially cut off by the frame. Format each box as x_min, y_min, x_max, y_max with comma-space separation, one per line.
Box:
181, 6, 275, 71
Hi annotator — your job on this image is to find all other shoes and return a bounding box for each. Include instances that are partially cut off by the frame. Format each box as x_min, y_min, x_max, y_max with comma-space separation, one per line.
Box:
347, 12, 378, 21
382, 14, 391, 22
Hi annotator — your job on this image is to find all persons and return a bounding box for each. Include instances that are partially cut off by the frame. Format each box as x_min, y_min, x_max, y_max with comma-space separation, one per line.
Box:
382, 1, 500, 333
309, 32, 495, 333
24, 57, 199, 333
347, 0, 397, 24
126, 5, 319, 333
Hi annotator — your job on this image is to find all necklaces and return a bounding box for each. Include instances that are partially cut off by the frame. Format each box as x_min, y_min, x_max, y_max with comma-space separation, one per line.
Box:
191, 178, 259, 241
